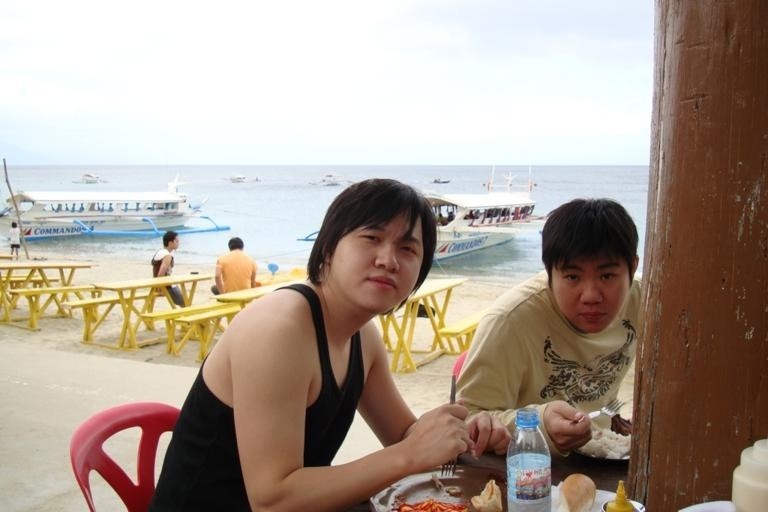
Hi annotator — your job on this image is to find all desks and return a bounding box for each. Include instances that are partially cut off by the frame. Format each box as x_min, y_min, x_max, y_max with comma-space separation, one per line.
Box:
379, 278, 464, 373
89, 274, 211, 350
209, 280, 306, 309
0, 263, 91, 323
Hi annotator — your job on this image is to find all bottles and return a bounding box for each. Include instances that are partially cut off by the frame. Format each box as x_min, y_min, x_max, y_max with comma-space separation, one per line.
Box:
506, 407, 553, 512
606, 480, 635, 512
728, 438, 768, 512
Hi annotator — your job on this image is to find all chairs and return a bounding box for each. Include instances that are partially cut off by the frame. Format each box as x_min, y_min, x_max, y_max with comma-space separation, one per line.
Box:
451, 350, 468, 403
70, 402, 182, 512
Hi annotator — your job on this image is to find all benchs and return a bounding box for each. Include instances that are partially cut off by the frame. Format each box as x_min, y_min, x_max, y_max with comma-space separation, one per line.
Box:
141, 302, 228, 354
9, 285, 94, 331
438, 317, 480, 356
0, 278, 59, 312
175, 304, 248, 363
60, 292, 168, 344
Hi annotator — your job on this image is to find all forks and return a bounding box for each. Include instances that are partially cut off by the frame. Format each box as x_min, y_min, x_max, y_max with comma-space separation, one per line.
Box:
440, 376, 459, 476
568, 396, 626, 427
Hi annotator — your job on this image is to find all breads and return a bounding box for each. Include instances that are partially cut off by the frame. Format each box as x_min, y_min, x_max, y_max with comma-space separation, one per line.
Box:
559, 471, 598, 512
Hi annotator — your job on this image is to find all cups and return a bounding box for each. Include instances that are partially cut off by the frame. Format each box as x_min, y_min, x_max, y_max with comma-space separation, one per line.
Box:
600, 499, 644, 512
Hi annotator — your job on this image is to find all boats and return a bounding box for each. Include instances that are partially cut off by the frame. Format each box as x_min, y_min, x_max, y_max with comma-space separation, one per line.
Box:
309, 174, 345, 187
67, 169, 113, 185
0, 191, 230, 243
427, 175, 454, 186
227, 173, 254, 185
294, 165, 549, 262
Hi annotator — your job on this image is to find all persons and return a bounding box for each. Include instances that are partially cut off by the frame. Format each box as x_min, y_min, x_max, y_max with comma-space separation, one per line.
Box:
9, 220, 21, 259
147, 180, 514, 510
4, 201, 172, 213
455, 199, 642, 456
433, 206, 533, 225
211, 236, 258, 295
151, 229, 187, 308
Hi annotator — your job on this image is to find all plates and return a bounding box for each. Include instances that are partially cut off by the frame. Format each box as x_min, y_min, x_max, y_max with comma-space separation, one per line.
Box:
571, 410, 630, 461
386, 464, 508, 512
